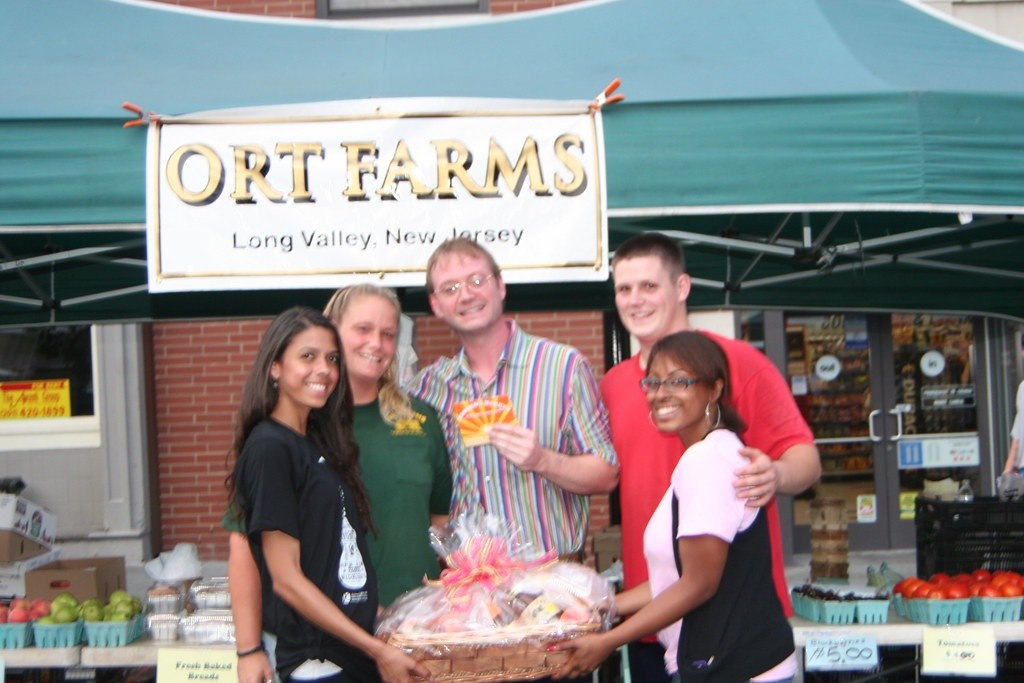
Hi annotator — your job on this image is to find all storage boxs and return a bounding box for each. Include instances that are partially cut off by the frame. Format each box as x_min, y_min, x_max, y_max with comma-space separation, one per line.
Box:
0, 556, 233, 656
790, 496, 1024, 625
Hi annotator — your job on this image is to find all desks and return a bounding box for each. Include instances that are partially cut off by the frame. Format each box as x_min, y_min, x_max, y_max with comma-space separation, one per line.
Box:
0, 629, 244, 683
786, 603, 1024, 683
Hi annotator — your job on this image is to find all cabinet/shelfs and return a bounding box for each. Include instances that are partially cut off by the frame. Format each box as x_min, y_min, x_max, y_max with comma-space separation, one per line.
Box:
805, 319, 976, 479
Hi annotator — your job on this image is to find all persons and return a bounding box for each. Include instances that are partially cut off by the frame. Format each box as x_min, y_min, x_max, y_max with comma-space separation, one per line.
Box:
405, 238, 620, 566
218, 283, 453, 683
1002, 378, 1024, 478
223, 305, 431, 683
546, 330, 802, 683
597, 234, 821, 683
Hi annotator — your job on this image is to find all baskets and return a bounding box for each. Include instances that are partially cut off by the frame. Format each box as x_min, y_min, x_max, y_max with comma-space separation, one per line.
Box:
375, 595, 611, 683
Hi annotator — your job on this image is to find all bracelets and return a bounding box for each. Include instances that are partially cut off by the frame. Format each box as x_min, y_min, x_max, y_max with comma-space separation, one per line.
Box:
237, 645, 263, 657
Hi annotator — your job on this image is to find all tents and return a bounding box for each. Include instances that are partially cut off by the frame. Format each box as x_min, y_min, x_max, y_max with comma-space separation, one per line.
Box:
1, 0, 1024, 329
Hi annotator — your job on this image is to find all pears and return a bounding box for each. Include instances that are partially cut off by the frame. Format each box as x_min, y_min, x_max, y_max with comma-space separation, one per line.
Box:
33, 589, 143, 625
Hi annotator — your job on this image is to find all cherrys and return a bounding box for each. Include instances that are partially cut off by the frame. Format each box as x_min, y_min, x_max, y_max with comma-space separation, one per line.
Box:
793, 578, 886, 601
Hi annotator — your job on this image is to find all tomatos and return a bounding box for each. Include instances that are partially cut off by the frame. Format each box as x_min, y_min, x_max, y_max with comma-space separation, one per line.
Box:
893, 567, 1024, 599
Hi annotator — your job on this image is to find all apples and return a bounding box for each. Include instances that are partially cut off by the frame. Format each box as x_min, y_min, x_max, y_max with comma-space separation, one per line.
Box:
0, 598, 49, 623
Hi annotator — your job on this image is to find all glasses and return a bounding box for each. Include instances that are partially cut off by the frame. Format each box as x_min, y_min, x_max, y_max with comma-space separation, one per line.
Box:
638, 376, 701, 392
431, 270, 498, 297
